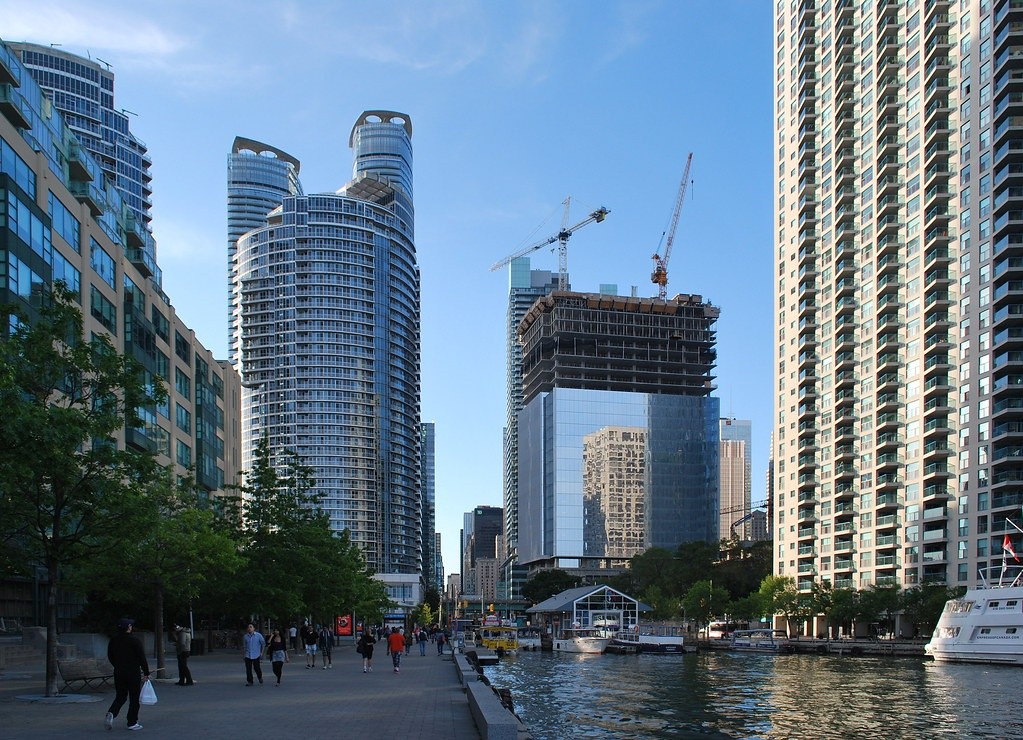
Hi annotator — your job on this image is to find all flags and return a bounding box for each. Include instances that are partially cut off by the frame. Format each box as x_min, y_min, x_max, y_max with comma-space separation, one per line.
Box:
1003, 551, 1007, 572
1003, 535, 1019, 562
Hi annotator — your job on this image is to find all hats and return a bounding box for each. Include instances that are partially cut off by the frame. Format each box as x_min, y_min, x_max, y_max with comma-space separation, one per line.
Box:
118, 618, 135, 630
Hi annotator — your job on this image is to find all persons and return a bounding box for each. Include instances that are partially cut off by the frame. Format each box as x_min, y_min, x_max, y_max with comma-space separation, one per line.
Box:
104, 619, 150, 730
400, 626, 448, 656
372, 625, 395, 656
388, 628, 406, 671
290, 623, 307, 650
243, 624, 266, 686
265, 627, 289, 686
304, 625, 319, 669
173, 622, 193, 686
484, 630, 500, 637
318, 626, 335, 669
358, 628, 377, 673
474, 632, 482, 648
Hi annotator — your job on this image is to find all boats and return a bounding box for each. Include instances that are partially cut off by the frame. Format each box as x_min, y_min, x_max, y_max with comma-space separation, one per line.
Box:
922, 517, 1023, 666
727, 628, 797, 654
610, 622, 686, 654
552, 621, 612, 654
471, 589, 542, 654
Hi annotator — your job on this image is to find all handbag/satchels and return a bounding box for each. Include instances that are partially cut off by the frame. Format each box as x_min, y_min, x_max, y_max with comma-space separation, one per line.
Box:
138, 679, 157, 705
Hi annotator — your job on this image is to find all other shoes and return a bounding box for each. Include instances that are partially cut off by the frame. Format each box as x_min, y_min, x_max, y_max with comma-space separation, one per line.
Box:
394, 667, 399, 672
276, 682, 281, 687
175, 680, 184, 685
421, 654, 425, 656
368, 668, 373, 672
183, 681, 193, 685
127, 722, 144, 731
103, 711, 114, 731
405, 653, 408, 656
311, 664, 316, 670
245, 682, 253, 686
258, 677, 263, 683
306, 665, 311, 669
363, 669, 367, 673
323, 665, 327, 670
437, 653, 444, 656
328, 663, 333, 668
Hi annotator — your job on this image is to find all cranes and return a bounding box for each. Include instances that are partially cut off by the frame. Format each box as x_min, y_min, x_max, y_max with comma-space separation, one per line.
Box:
487, 195, 612, 292
648, 150, 697, 299
